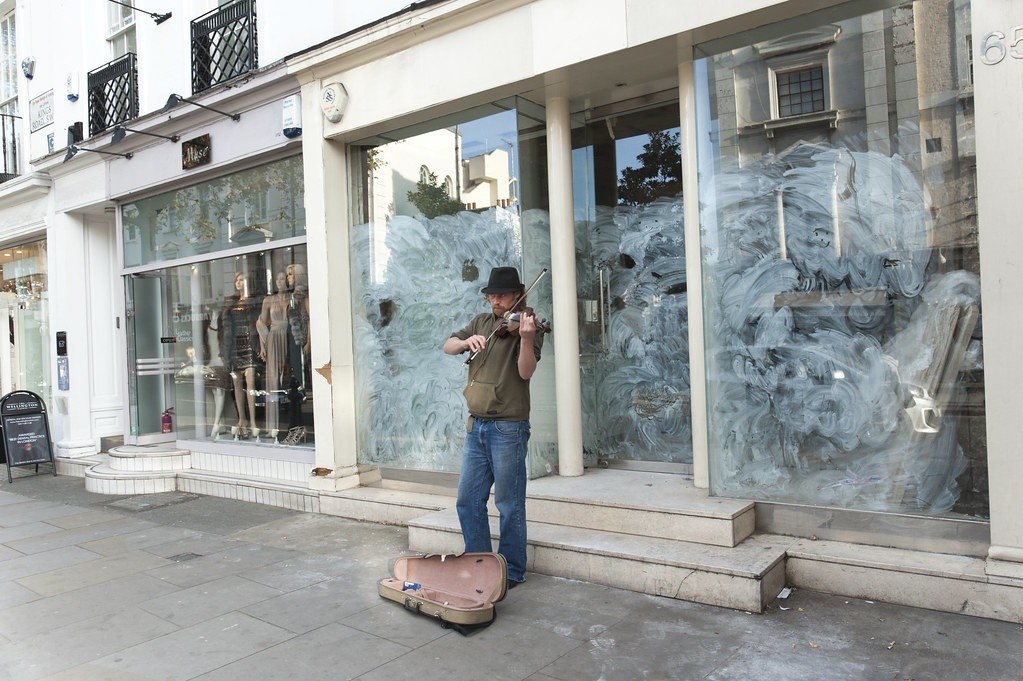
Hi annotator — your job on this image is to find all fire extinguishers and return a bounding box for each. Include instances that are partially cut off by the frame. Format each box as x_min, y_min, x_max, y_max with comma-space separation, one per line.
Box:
161, 407, 174, 433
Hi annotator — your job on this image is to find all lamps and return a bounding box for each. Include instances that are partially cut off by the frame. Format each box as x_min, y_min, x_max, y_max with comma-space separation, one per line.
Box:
62, 145, 134, 163
158, 94, 240, 121
110, 124, 181, 145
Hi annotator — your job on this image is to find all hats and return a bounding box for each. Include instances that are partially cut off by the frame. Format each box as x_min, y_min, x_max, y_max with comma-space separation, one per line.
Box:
480, 267, 525, 294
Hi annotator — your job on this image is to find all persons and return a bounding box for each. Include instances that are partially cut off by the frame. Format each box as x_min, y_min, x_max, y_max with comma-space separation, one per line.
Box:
203, 264, 314, 437
442, 267, 545, 592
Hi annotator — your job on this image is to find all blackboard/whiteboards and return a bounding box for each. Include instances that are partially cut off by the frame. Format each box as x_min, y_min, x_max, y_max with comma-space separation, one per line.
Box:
0, 390, 53, 467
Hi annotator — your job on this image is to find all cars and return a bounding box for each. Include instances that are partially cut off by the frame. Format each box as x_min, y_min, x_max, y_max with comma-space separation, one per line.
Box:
173, 355, 305, 420
636, 323, 945, 500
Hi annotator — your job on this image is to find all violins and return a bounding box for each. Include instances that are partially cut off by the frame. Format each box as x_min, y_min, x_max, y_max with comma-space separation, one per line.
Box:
491, 306, 551, 336
